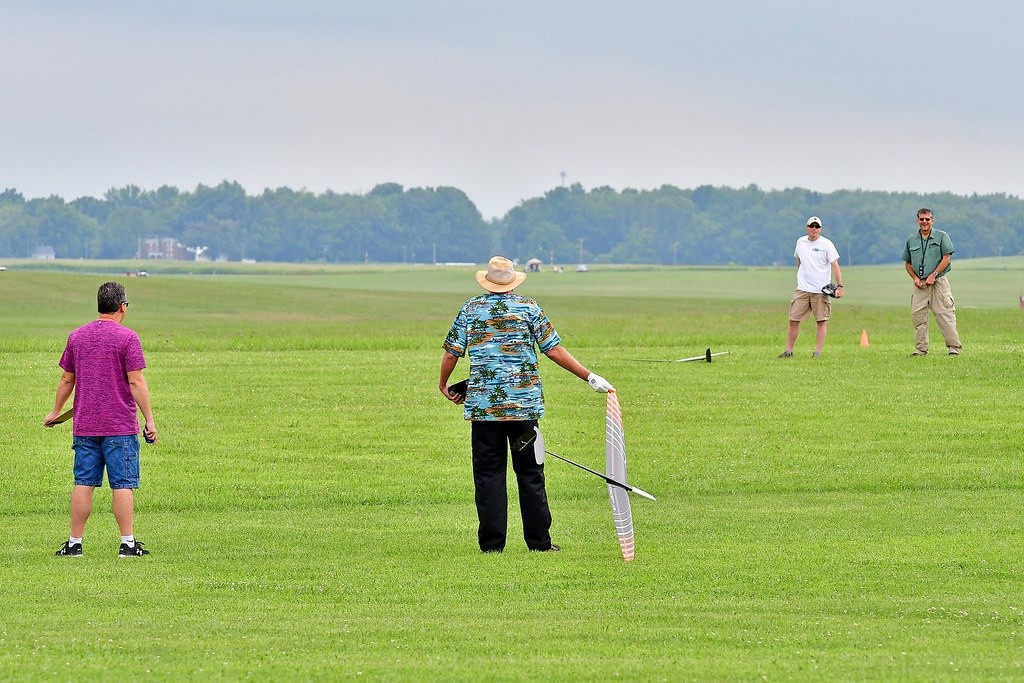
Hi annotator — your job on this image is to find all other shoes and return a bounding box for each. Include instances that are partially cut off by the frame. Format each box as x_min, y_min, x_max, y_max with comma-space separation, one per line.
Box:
779, 352, 792, 358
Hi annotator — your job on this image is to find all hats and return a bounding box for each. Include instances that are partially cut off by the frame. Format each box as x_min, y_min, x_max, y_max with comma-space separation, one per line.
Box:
807, 216, 822, 227
475, 256, 527, 293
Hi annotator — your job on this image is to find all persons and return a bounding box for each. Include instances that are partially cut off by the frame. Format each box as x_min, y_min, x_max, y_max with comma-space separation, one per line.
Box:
901, 208, 962, 358
45, 282, 157, 559
779, 216, 843, 358
438, 255, 615, 552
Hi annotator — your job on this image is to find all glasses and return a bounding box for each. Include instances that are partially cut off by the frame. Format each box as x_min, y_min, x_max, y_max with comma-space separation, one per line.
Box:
809, 225, 820, 228
122, 301, 129, 307
919, 218, 930, 221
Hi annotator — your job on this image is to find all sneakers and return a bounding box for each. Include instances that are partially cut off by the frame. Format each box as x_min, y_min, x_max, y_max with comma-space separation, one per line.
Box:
119, 540, 150, 558
56, 540, 82, 557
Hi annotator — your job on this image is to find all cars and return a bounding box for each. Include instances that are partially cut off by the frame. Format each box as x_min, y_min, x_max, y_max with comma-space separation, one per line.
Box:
576, 265, 587, 272
0, 266, 7, 271
138, 269, 148, 277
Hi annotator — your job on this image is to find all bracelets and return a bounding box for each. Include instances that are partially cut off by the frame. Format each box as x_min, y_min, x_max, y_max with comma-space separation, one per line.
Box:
933, 271, 938, 276
837, 284, 843, 287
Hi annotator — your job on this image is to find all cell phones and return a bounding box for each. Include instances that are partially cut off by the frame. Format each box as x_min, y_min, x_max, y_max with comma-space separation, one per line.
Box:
143, 430, 154, 443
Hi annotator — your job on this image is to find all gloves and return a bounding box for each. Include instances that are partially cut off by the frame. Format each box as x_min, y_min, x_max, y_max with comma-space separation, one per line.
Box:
587, 372, 616, 393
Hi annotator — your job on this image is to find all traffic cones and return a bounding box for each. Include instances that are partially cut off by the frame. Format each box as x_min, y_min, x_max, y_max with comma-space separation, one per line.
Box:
860, 329, 869, 346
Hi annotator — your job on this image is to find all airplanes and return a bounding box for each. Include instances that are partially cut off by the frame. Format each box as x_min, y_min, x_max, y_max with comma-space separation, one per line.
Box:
519, 390, 657, 561
676, 348, 730, 363
